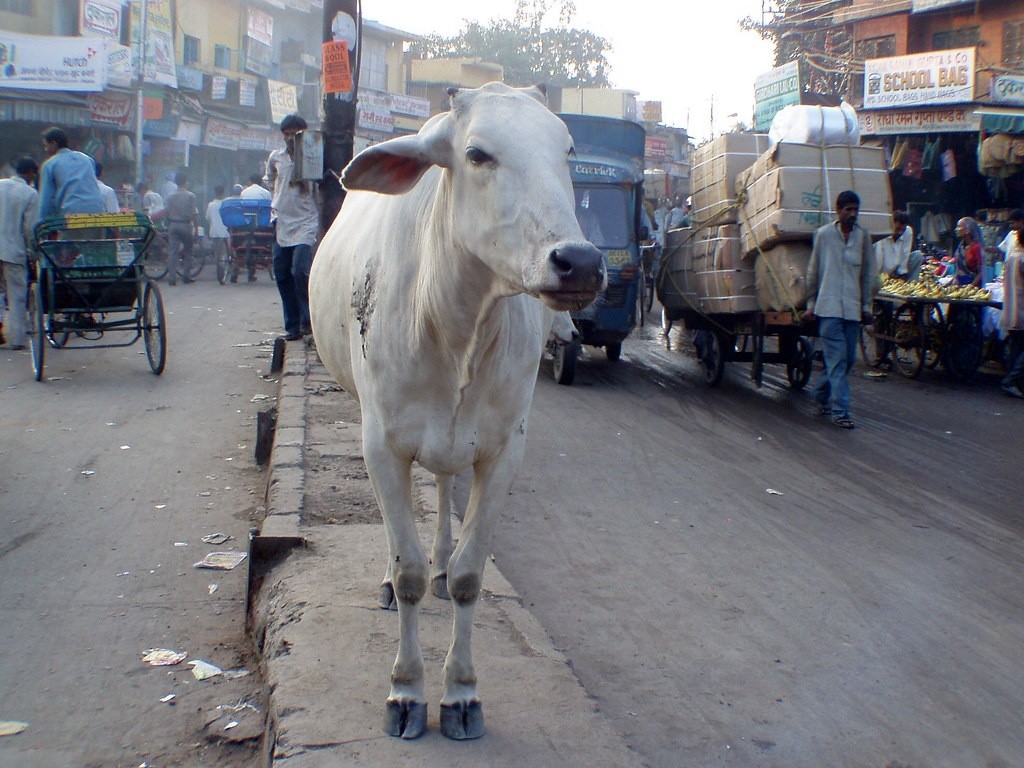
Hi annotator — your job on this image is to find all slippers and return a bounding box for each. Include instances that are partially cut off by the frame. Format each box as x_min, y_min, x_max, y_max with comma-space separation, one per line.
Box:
1001, 384, 1024, 399
897, 356, 912, 364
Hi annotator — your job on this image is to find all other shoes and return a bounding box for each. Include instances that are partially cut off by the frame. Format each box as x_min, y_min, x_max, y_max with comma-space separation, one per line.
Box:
299, 324, 312, 335
813, 391, 831, 415
169, 281, 176, 285
284, 328, 303, 341
873, 357, 895, 371
183, 276, 195, 283
12, 344, 22, 350
248, 276, 257, 282
0, 334, 6, 345
832, 415, 854, 428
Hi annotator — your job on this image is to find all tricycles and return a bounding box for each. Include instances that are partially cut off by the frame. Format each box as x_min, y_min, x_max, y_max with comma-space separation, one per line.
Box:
215, 198, 276, 286
25, 211, 167, 382
637, 243, 662, 328
143, 208, 206, 280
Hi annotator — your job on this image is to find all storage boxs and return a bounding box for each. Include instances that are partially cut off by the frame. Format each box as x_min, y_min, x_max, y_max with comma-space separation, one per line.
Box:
691, 221, 758, 317
682, 131, 772, 225
656, 226, 692, 316
736, 139, 898, 261
219, 198, 275, 231
752, 238, 813, 311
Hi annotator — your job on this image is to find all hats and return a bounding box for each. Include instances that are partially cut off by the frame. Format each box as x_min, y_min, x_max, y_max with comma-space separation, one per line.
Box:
684, 196, 692, 206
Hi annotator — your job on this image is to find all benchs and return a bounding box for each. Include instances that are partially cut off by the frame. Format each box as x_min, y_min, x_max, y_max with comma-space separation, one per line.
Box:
32, 237, 144, 314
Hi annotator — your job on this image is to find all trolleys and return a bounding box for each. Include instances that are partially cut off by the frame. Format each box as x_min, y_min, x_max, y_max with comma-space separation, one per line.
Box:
683, 312, 865, 390
857, 293, 1003, 379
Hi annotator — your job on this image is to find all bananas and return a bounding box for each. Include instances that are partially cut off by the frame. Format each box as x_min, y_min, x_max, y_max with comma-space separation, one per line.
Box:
879, 266, 993, 300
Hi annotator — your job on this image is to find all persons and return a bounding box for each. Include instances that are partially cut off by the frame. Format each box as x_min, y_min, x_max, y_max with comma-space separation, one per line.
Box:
261, 115, 318, 341
94, 162, 120, 243
805, 190, 873, 428
641, 188, 692, 286
0, 153, 39, 350
872, 211, 922, 370
40, 127, 108, 323
137, 173, 272, 287
573, 187, 605, 245
975, 206, 1024, 397
934, 217, 986, 371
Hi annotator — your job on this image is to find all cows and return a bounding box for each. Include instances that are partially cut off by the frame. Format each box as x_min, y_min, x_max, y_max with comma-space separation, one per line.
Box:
308, 81, 608, 740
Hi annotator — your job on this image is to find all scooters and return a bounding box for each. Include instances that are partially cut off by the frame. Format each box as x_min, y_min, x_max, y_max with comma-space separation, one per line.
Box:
545, 113, 649, 384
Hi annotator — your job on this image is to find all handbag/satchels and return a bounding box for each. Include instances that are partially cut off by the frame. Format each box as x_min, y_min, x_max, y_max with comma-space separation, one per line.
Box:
890, 136, 909, 171
921, 139, 943, 180
940, 146, 978, 195
903, 134, 927, 181
